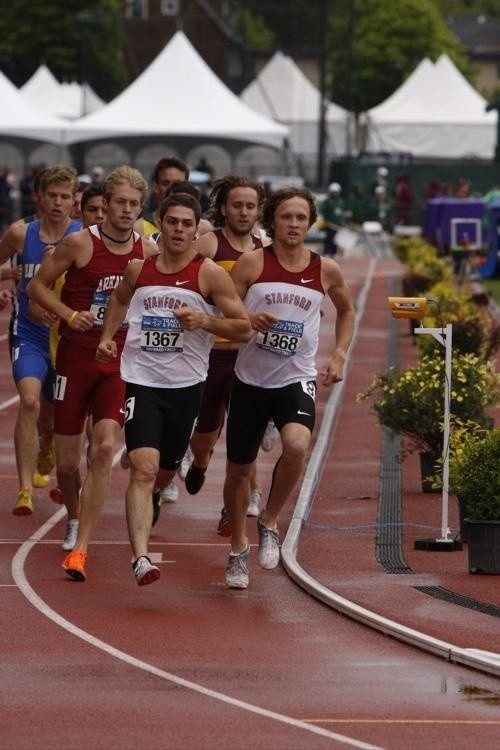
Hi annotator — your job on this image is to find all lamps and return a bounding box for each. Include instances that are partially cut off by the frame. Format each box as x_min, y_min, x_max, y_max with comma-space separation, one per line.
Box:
363, 221, 394, 256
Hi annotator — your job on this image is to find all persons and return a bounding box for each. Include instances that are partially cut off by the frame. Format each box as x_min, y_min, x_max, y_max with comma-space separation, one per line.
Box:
359, 162, 487, 235
134, 155, 217, 243
147, 180, 211, 251
317, 180, 352, 257
184, 173, 272, 539
25, 164, 156, 582
69, 179, 91, 223
25, 185, 107, 505
224, 186, 356, 592
1, 168, 89, 519
0, 286, 14, 313
7, 169, 56, 487
94, 193, 253, 587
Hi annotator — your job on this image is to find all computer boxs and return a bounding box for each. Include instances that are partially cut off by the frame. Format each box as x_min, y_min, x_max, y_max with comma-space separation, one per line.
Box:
387, 296, 427, 321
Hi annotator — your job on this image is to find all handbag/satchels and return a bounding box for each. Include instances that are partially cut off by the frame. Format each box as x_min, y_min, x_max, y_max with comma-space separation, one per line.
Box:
66, 308, 81, 325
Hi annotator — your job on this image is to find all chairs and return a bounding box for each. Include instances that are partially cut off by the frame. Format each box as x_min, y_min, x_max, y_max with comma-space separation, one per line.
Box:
132, 555, 161, 586
246, 488, 261, 516
162, 481, 178, 501
178, 450, 191, 480
13, 490, 33, 516
61, 549, 88, 580
256, 519, 280, 570
33, 449, 54, 487
62, 519, 80, 550
262, 422, 275, 451
225, 537, 250, 589
216, 507, 231, 537
185, 462, 207, 494
121, 449, 130, 468
153, 492, 161, 526
